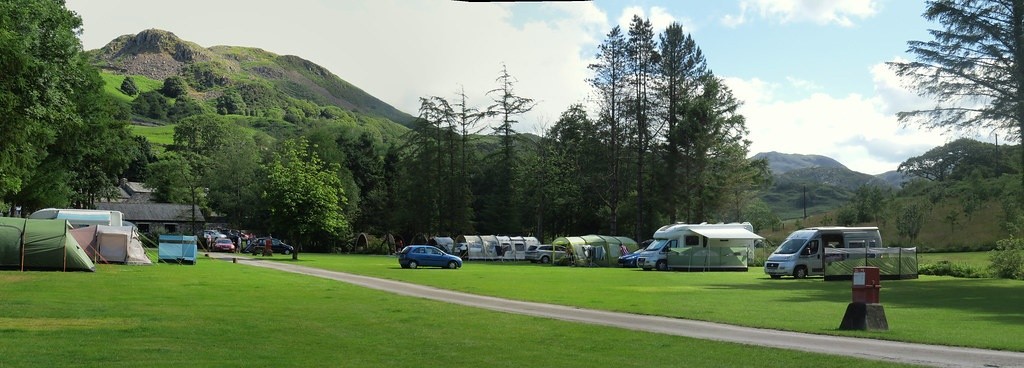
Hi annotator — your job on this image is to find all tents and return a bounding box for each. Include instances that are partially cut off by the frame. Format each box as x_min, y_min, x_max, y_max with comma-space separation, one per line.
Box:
69, 225, 151, 263
0, 217, 95, 272
452, 235, 540, 261
553, 234, 639, 267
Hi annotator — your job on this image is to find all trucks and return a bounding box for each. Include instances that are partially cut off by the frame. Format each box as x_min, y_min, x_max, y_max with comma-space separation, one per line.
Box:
636, 221, 765, 272
763, 226, 883, 281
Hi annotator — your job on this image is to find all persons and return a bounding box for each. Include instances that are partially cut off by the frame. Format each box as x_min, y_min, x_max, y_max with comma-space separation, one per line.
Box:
246, 233, 256, 245
233, 231, 241, 253
208, 232, 211, 247
265, 237, 271, 250
619, 243, 628, 254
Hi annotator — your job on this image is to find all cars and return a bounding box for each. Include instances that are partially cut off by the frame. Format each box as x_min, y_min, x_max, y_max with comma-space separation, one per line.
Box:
617, 246, 647, 268
250, 236, 294, 255
203, 228, 254, 253
399, 245, 462, 269
524, 243, 571, 264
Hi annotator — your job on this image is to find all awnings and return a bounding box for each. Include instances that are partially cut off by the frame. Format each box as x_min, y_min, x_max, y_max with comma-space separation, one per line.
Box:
689, 228, 764, 239
